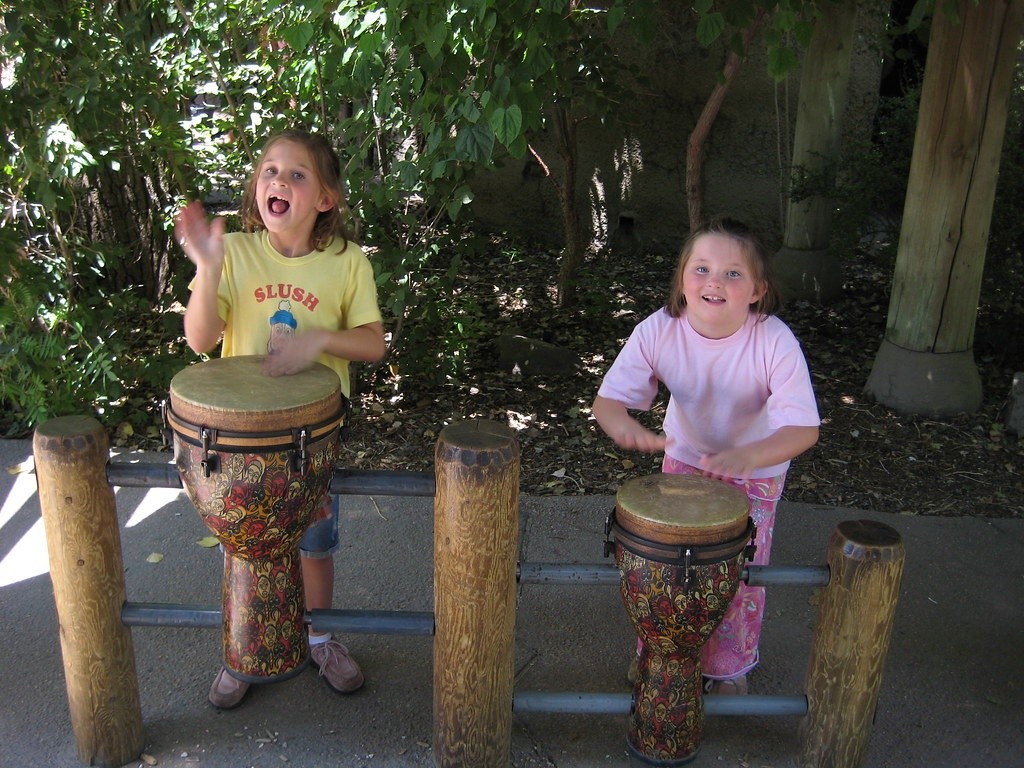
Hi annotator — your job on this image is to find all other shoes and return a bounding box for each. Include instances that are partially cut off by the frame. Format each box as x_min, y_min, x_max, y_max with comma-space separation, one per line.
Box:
704, 673, 749, 695
627, 654, 642, 683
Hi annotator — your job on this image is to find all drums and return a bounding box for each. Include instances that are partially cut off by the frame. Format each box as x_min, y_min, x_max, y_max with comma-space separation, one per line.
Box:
602, 474, 758, 766
163, 355, 351, 686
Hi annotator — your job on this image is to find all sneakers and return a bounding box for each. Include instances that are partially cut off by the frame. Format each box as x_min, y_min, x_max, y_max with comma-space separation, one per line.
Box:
309, 634, 364, 695
207, 665, 257, 709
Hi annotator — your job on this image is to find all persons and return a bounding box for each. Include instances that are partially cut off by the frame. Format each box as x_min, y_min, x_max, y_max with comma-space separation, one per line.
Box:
174, 129, 384, 706
593, 218, 821, 697
